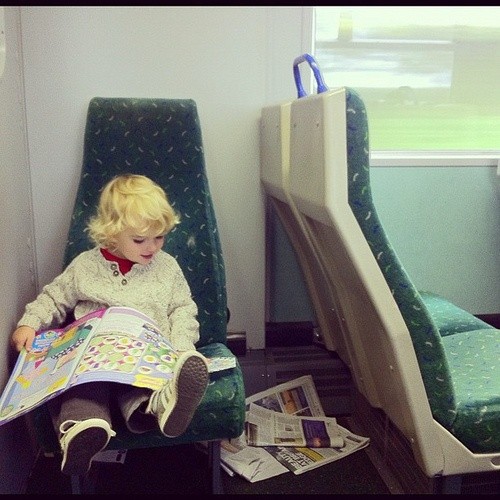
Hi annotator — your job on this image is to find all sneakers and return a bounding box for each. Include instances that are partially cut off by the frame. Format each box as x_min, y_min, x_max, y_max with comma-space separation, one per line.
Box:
59, 418, 116, 476
145, 350, 209, 437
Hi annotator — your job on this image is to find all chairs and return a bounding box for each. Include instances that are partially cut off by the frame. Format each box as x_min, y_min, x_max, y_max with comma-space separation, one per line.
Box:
33, 98, 247, 500
258, 88, 500, 495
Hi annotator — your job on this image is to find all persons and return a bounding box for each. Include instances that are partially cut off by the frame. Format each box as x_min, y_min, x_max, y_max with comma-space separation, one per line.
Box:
7, 172, 209, 477
